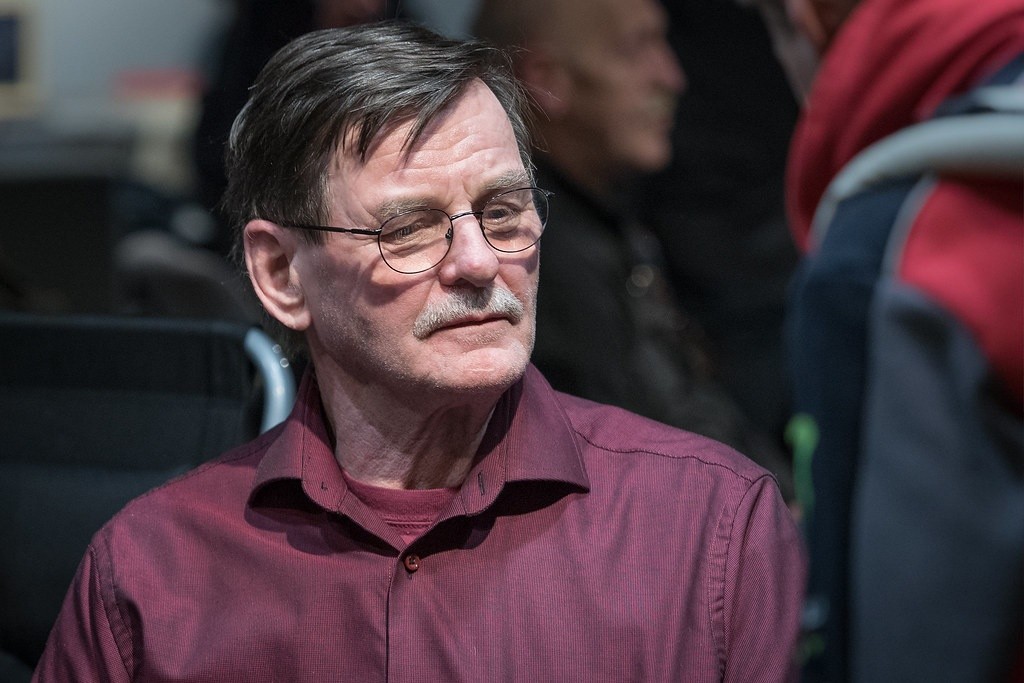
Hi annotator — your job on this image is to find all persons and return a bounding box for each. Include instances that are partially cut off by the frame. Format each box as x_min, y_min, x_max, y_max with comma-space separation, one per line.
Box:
186, 1, 809, 476
26, 19, 807, 683
766, 1, 1023, 683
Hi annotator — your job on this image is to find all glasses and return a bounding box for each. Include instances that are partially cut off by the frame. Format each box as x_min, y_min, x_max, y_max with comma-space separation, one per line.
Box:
274, 188, 556, 276
